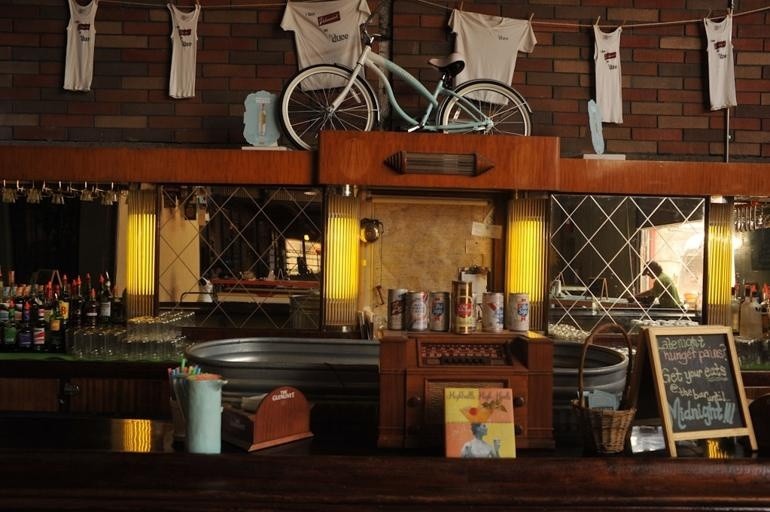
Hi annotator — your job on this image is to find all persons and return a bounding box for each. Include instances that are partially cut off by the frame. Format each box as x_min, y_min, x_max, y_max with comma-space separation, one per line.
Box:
629, 262, 677, 307
461, 422, 500, 460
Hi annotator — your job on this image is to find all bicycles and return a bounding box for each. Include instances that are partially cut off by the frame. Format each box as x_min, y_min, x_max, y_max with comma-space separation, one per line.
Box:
280, 17, 534, 160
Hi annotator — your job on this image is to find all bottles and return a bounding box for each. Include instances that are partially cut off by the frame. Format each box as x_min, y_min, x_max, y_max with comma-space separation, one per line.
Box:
0, 267, 115, 353
730, 282, 770, 339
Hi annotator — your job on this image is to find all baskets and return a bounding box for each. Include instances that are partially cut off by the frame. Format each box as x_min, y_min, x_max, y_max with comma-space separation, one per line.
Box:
571, 325, 636, 454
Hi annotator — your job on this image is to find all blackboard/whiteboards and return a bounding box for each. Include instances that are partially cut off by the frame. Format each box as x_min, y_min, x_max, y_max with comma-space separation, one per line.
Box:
647, 325, 753, 440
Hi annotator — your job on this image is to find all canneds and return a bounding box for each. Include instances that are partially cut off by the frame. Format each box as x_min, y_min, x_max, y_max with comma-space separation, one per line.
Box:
388, 288, 408, 330
409, 291, 429, 330
482, 292, 504, 332
429, 291, 450, 332
452, 280, 473, 335
510, 293, 530, 331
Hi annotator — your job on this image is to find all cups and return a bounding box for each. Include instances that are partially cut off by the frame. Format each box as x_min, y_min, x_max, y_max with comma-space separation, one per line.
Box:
65, 307, 198, 360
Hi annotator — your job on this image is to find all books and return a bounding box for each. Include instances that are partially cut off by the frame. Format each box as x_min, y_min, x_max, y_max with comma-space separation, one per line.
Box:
444, 387, 515, 459
